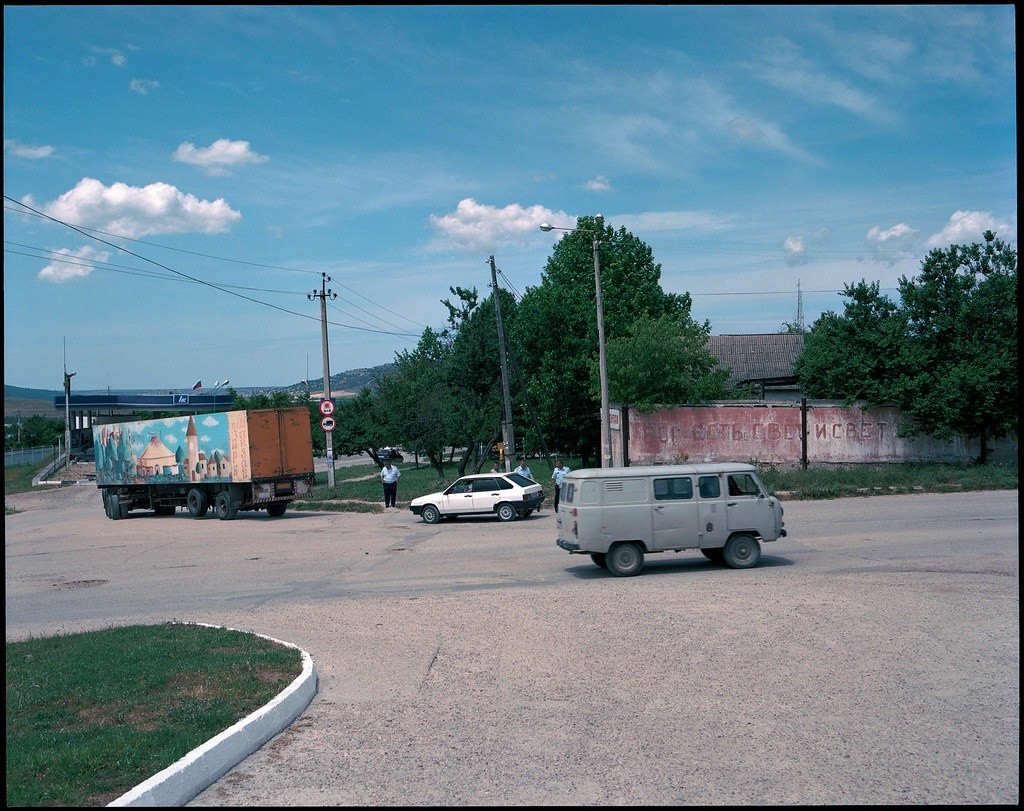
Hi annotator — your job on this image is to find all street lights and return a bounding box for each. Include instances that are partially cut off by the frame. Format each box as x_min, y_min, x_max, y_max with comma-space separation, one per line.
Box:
539, 213, 612, 468
213, 380, 229, 413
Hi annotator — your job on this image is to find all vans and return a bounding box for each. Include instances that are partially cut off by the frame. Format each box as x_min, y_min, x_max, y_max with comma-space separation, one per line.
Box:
555, 462, 788, 577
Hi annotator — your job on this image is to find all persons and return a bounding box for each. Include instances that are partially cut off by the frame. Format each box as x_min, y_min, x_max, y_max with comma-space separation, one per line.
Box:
381, 462, 401, 509
551, 459, 571, 513
490, 462, 502, 473
467, 483, 473, 492
514, 460, 534, 480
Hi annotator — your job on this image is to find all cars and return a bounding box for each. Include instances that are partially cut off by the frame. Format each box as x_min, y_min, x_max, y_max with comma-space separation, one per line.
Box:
409, 471, 546, 525
375, 448, 404, 463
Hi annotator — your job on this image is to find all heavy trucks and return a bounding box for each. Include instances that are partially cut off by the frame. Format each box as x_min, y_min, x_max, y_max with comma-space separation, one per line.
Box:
90, 407, 317, 521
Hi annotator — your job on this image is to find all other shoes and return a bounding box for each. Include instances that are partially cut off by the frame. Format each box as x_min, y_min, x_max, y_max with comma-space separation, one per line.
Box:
555, 510, 558, 513
386, 506, 389, 508
392, 505, 395, 507
